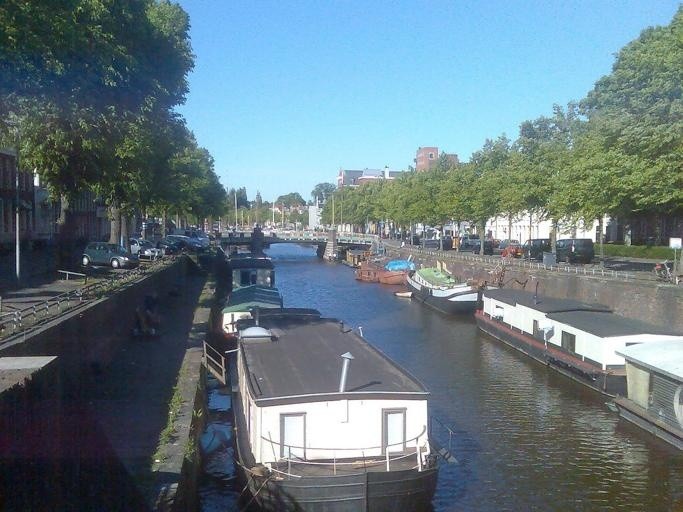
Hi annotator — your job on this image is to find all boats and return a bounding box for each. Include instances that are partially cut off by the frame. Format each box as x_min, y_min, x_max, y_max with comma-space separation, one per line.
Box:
341, 248, 370, 269
355, 260, 382, 282
377, 260, 415, 285
228, 306, 458, 512
217, 283, 284, 344
476, 283, 666, 398
610, 336, 683, 449
224, 250, 276, 292
405, 262, 485, 316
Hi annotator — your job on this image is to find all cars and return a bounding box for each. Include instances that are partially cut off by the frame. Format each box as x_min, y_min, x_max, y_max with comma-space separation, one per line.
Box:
498, 239, 524, 258
81, 242, 140, 269
461, 239, 492, 256
129, 230, 211, 259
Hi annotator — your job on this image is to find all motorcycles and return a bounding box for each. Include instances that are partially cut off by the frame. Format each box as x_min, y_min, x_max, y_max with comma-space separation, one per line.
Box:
654, 260, 673, 280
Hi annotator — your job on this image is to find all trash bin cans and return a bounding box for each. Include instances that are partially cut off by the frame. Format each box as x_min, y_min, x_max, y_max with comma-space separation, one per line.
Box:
543, 252, 553, 268
409, 235, 417, 245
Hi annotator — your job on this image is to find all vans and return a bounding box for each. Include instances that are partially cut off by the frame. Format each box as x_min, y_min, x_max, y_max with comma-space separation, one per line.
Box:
521, 239, 595, 264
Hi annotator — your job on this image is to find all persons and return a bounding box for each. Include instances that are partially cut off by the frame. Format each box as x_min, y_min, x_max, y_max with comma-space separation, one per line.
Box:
145, 304, 161, 330
